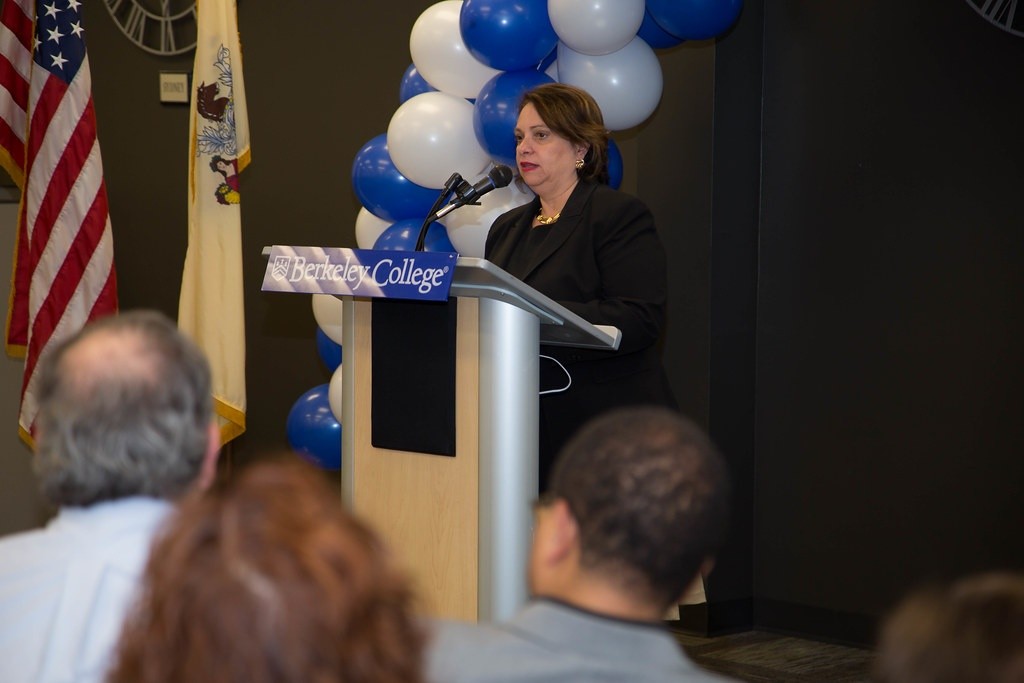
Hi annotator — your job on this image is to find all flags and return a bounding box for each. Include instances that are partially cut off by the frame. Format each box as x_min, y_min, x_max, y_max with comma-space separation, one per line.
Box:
0, 0, 119, 449
177, 0, 253, 447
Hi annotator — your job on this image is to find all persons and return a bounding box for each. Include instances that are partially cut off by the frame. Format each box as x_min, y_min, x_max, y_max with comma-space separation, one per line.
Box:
484, 82, 669, 496
102, 481, 434, 683
0, 308, 219, 683
878, 573, 1024, 683
422, 405, 743, 683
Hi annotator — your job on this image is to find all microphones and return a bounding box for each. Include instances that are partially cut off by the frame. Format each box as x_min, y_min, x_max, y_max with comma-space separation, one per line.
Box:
428, 165, 513, 223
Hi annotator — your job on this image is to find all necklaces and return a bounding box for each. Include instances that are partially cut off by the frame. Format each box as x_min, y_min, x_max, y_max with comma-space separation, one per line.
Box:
537, 207, 560, 225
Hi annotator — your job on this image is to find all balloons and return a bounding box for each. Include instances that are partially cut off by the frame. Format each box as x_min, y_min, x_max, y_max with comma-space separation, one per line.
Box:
288, 0, 748, 467
